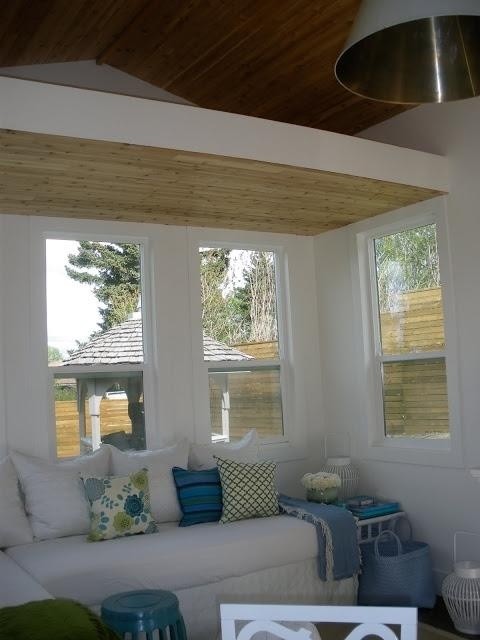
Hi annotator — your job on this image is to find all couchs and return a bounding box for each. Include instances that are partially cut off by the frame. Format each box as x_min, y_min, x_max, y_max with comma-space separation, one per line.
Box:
1, 428, 361, 640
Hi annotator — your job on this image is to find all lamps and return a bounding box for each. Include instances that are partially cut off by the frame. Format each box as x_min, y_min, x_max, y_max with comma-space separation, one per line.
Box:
334, 1, 480, 105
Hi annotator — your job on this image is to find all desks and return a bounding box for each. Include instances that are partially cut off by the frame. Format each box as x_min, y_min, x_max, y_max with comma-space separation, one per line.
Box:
354, 511, 407, 566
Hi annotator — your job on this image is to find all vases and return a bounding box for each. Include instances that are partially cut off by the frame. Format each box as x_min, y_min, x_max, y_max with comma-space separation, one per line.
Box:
307, 488, 339, 505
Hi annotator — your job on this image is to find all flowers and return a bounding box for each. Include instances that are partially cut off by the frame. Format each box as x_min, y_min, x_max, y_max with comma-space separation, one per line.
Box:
300, 470, 341, 496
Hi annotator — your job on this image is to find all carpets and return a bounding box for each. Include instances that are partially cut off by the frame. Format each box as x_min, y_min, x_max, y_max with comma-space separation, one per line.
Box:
315, 621, 468, 640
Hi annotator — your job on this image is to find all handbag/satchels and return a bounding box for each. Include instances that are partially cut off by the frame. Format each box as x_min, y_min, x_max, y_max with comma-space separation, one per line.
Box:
359, 516, 436, 609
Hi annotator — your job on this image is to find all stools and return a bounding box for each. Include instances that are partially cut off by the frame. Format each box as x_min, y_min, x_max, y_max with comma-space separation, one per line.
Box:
101, 589, 188, 640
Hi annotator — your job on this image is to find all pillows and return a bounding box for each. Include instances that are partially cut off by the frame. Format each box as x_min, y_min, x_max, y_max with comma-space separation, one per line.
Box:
0, 429, 282, 550
